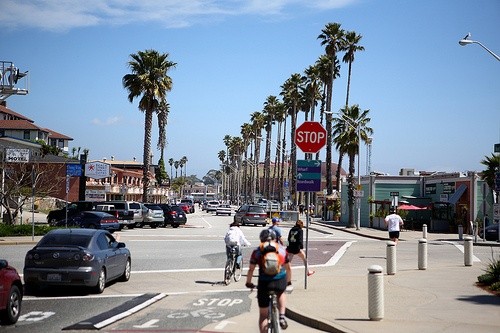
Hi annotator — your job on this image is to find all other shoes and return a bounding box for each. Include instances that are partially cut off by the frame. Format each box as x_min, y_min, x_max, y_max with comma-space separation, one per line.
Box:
278, 318, 288, 329
308, 270, 315, 276
235, 262, 241, 269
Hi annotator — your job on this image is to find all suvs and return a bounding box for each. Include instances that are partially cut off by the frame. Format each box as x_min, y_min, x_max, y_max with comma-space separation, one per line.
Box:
155, 204, 187, 228
135, 202, 165, 229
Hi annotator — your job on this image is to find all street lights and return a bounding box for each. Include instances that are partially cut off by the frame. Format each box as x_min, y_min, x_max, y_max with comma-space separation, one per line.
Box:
322, 110, 361, 230
257, 136, 289, 202
220, 164, 240, 207
233, 154, 256, 202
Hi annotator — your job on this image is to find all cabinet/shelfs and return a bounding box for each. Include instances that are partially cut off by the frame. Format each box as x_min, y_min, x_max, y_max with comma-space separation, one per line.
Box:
3, 209, 15, 222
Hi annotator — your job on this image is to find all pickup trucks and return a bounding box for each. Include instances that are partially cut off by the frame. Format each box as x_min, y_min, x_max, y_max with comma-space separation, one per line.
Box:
47, 201, 135, 231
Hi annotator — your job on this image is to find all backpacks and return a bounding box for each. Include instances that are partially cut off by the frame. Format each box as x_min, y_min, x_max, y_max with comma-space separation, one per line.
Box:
259, 245, 283, 274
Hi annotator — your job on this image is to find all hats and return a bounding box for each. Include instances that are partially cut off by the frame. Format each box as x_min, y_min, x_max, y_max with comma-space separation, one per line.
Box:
271, 217, 280, 222
259, 229, 276, 242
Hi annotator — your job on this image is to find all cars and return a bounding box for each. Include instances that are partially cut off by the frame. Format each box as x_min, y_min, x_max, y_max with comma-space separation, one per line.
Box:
0, 259, 24, 325
56, 211, 120, 233
23, 229, 132, 297
179, 196, 292, 226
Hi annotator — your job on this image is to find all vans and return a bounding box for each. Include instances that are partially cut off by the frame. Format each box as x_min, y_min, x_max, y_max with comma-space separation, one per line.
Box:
93, 201, 143, 229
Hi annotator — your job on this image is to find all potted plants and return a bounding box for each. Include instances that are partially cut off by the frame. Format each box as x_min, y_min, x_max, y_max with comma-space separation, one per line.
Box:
335, 213, 340, 221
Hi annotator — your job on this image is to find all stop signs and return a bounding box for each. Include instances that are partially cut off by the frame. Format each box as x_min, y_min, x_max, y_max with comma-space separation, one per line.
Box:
294, 121, 327, 153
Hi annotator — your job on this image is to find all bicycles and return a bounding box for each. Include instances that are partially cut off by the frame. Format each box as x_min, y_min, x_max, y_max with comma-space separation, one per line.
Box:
247, 282, 291, 333
225, 244, 243, 284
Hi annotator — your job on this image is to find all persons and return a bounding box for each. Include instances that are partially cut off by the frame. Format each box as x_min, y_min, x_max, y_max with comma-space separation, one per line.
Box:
224, 221, 250, 284
183, 196, 328, 222
247, 229, 292, 332
268, 218, 284, 246
288, 220, 315, 276
385, 208, 404, 244
